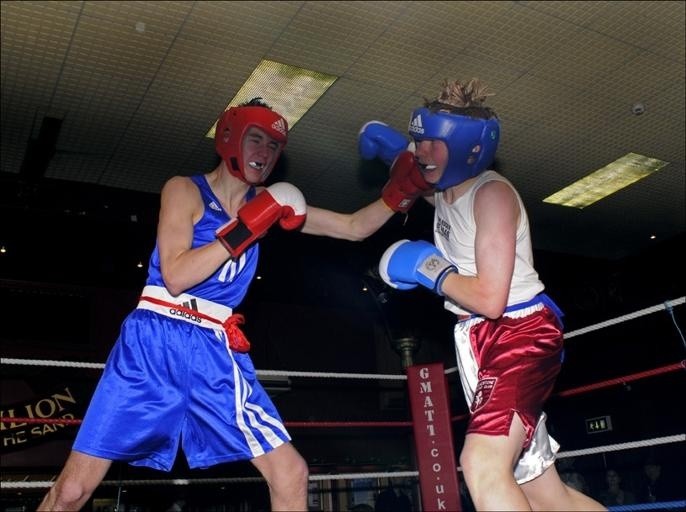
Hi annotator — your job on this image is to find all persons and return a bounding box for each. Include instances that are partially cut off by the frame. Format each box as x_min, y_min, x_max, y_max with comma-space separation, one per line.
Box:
560, 458, 680, 512
35, 95, 436, 511
356, 78, 611, 512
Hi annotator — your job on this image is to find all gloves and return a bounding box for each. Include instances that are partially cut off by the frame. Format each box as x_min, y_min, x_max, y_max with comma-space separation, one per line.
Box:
215, 182, 307, 256
379, 239, 458, 299
214, 106, 288, 182
359, 120, 437, 215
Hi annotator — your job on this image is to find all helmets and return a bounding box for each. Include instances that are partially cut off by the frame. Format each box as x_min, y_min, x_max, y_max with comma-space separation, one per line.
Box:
409, 108, 501, 191
